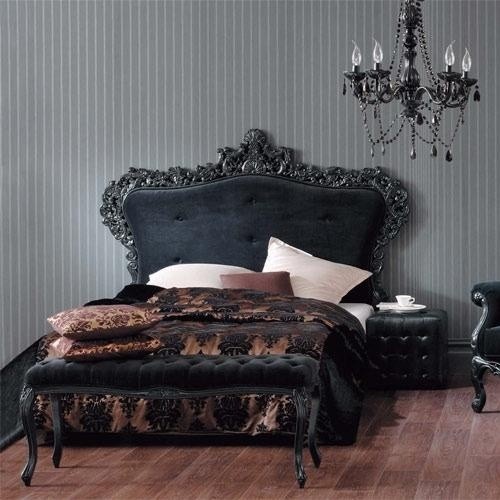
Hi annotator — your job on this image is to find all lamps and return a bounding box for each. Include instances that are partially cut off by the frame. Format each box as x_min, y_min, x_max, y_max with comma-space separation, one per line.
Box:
341, 1, 480, 162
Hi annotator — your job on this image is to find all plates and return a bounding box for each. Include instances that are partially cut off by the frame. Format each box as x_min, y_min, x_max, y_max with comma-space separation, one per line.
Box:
388, 303, 427, 315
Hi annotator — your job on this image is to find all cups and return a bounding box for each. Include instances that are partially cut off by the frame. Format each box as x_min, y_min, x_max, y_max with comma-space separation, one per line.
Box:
395, 294, 416, 307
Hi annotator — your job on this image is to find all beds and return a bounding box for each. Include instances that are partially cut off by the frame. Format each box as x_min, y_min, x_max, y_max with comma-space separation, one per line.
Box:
15, 127, 411, 489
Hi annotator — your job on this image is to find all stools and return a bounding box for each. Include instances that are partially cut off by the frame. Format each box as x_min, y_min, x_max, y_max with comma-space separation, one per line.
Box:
469, 281, 500, 413
368, 310, 448, 388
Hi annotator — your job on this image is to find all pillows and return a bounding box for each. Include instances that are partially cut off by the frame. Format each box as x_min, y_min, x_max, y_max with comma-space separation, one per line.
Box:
48, 333, 165, 360
147, 263, 255, 288
48, 304, 164, 339
220, 271, 294, 296
262, 237, 372, 305
